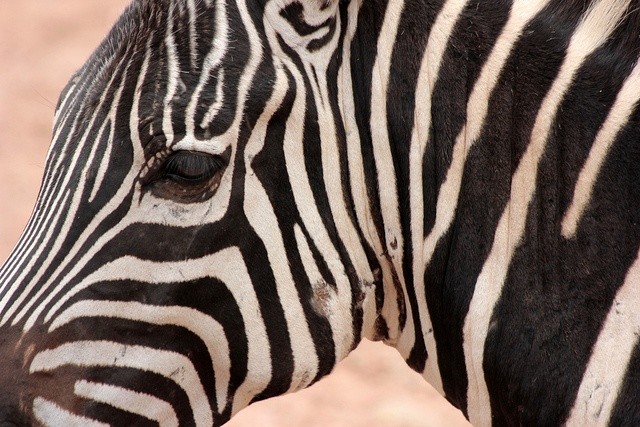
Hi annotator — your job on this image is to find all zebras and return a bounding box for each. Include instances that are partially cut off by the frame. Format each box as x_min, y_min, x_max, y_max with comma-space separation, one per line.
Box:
0, 0, 640, 427
